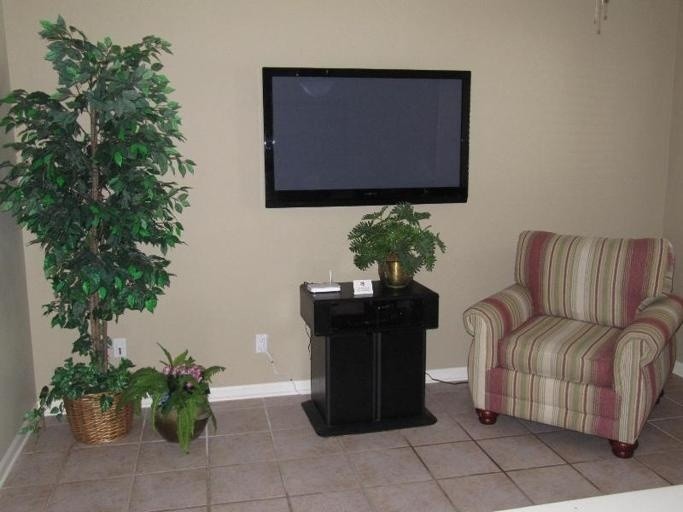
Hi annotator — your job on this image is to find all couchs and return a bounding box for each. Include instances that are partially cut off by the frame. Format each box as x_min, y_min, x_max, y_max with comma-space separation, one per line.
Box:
462, 230, 683, 459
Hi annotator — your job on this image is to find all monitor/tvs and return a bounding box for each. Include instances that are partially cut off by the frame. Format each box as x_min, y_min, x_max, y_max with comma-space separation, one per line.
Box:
262, 67, 471, 208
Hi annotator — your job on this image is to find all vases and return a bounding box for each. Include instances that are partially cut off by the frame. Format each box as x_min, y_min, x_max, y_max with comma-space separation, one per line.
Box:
152, 393, 209, 443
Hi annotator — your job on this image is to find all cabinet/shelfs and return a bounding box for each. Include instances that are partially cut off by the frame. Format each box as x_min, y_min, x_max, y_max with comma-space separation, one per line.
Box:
300, 278, 438, 437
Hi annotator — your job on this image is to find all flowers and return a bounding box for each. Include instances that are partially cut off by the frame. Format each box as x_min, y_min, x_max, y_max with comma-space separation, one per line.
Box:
116, 339, 229, 455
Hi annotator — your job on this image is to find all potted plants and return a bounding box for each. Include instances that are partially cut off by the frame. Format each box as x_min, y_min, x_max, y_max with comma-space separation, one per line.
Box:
346, 200, 446, 290
1, 17, 198, 444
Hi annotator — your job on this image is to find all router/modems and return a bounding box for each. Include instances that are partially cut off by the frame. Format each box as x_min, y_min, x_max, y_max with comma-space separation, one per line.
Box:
306, 270, 341, 294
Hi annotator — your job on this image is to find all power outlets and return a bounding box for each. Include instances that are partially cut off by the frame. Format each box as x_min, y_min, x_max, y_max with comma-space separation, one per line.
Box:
255, 335, 268, 354
